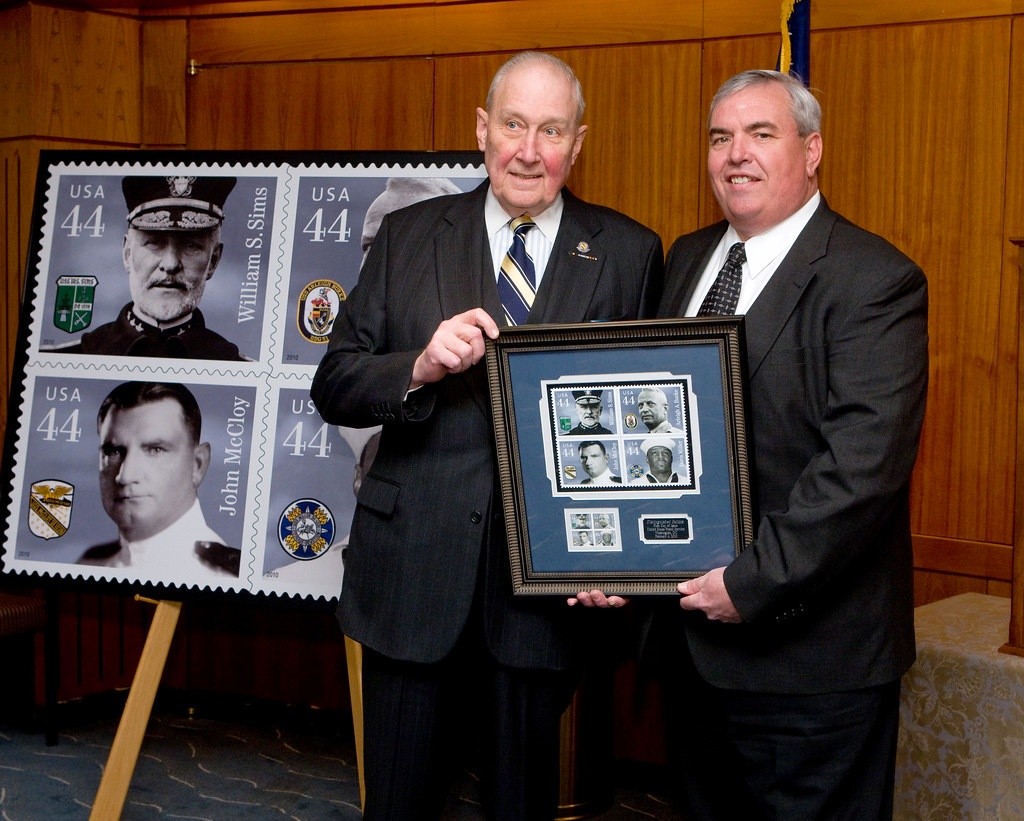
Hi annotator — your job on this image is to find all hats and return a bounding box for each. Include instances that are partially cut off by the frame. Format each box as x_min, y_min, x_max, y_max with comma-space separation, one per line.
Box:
641, 436, 675, 455
121, 175, 238, 231
572, 390, 603, 405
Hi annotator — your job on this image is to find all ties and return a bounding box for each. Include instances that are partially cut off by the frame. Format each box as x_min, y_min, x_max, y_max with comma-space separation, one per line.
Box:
495, 214, 537, 326
696, 242, 747, 318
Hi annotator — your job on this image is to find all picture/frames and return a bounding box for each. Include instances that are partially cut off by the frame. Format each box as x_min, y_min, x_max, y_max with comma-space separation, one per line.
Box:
0, 149, 487, 609
481, 315, 763, 616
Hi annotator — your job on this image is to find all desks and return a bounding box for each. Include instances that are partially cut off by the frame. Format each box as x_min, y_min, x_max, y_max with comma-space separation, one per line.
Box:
890, 588, 1023, 821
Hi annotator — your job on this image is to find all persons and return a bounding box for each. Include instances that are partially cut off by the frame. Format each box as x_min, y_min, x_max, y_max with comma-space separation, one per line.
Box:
638, 386, 683, 433
358, 177, 462, 278
579, 531, 594, 546
73, 382, 242, 579
628, 438, 689, 483
309, 50, 667, 821
597, 514, 614, 529
576, 514, 590, 529
265, 425, 383, 593
576, 441, 621, 484
633, 70, 928, 820
567, 390, 612, 434
39, 175, 258, 362
598, 531, 615, 546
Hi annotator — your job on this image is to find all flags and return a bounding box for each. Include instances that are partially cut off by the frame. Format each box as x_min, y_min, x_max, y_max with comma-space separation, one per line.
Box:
775, 0, 811, 90
